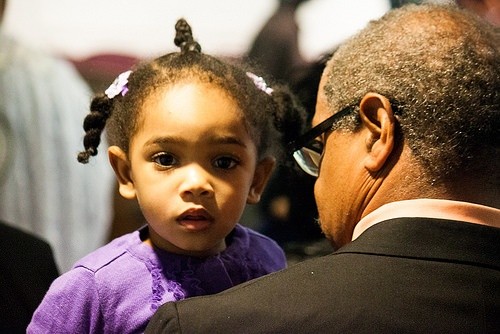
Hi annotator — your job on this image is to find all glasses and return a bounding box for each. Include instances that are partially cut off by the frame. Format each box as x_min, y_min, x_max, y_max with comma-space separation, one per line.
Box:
287, 102, 361, 180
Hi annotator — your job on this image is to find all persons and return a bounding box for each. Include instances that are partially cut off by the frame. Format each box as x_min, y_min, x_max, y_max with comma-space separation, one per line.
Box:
243, 0, 329, 248
27, 19, 308, 334
0, 222, 60, 334
144, 0, 500, 334
